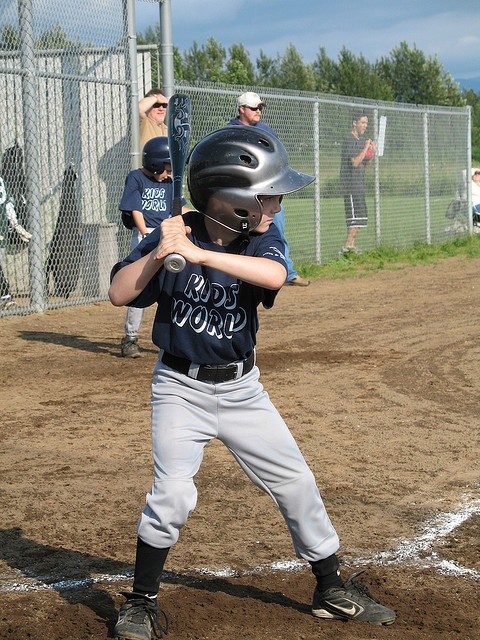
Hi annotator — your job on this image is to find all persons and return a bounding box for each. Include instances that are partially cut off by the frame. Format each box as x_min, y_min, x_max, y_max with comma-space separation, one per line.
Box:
227, 92, 311, 287
339, 111, 377, 252
108, 125, 396, 639
138, 88, 168, 152
0, 175, 32, 302
462, 167, 480, 231
120, 136, 195, 358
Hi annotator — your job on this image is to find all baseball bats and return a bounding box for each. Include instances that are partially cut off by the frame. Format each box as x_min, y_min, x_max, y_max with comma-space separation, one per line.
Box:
164, 93, 190, 275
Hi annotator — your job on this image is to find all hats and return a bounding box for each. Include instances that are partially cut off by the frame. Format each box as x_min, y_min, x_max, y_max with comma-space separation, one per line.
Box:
237, 92, 268, 108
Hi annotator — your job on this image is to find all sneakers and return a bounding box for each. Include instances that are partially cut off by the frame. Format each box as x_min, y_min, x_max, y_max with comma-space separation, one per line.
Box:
288, 275, 309, 287
312, 586, 395, 626
3, 295, 17, 309
115, 591, 168, 640
121, 337, 140, 357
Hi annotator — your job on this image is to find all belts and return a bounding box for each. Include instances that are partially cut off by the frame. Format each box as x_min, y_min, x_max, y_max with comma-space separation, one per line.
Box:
162, 350, 255, 383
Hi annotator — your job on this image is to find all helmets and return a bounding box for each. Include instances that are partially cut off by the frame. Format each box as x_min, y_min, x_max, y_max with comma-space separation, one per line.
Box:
142, 137, 171, 174
187, 126, 316, 246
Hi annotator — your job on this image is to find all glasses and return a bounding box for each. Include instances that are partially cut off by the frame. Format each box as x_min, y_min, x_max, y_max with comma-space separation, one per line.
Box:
475, 171, 480, 175
246, 106, 263, 111
152, 103, 167, 109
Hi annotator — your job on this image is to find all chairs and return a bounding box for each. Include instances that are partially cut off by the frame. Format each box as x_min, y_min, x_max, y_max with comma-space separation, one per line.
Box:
453, 182, 480, 230
